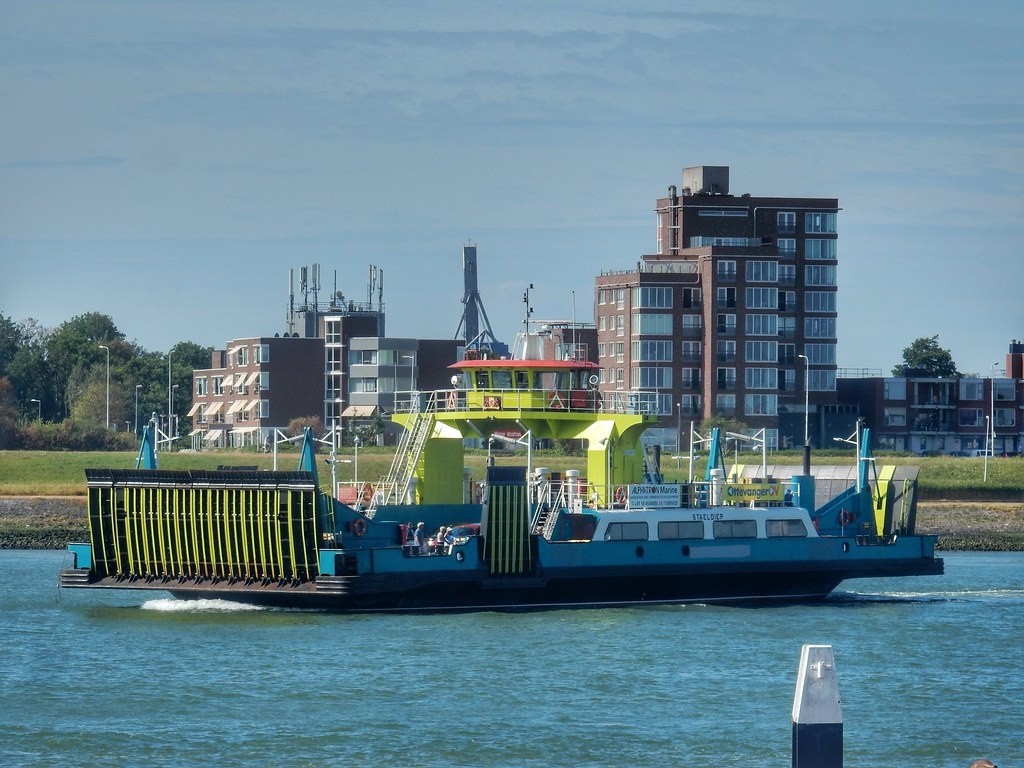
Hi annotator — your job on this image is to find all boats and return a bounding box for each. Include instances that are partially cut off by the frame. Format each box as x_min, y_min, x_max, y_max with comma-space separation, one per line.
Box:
56, 325, 948, 632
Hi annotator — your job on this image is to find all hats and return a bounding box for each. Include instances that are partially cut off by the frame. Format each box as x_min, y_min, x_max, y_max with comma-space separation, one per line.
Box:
447, 527, 452, 531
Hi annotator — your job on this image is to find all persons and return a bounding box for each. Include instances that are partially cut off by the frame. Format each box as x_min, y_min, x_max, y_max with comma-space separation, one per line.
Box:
437, 526, 453, 553
401, 521, 425, 554
785, 490, 793, 507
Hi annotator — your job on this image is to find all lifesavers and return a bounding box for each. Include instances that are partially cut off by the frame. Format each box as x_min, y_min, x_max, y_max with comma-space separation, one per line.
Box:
548, 391, 566, 408
363, 484, 373, 501
589, 375, 598, 385
353, 518, 366, 536
840, 510, 849, 526
616, 488, 626, 503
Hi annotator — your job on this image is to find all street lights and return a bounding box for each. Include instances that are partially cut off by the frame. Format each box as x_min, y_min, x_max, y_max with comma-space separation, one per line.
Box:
991, 362, 999, 456
799, 354, 810, 445
98, 345, 110, 430
30, 398, 41, 422
